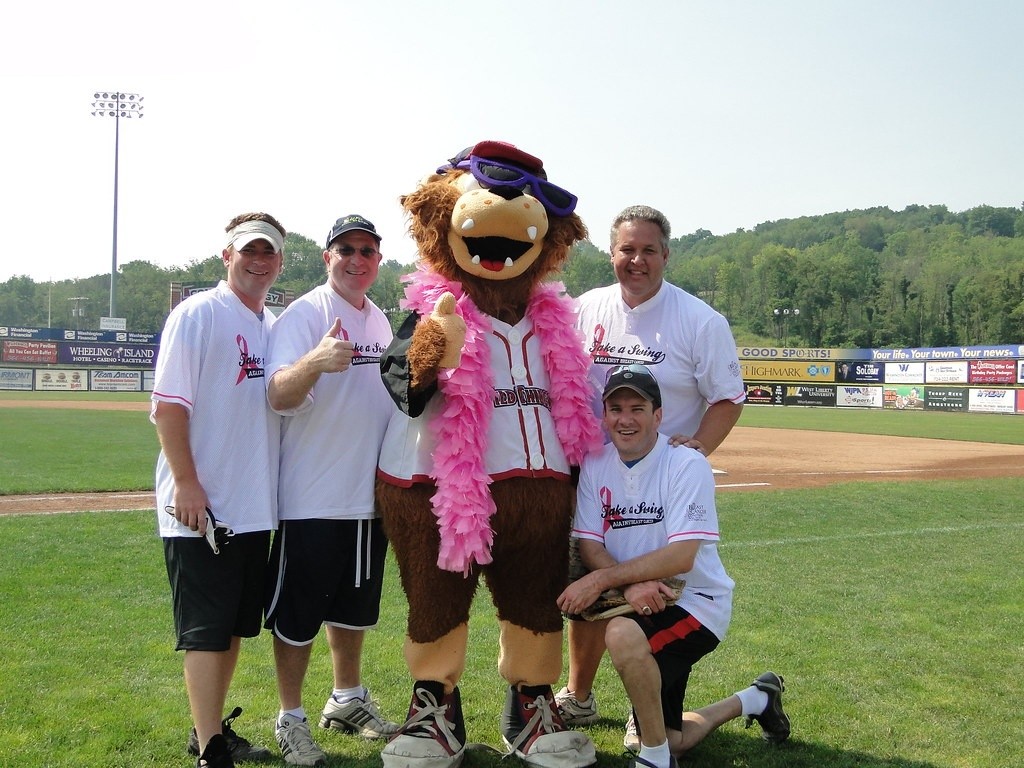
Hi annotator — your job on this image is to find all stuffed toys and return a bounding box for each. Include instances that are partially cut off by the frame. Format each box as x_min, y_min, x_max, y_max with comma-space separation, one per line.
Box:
376, 140, 603, 768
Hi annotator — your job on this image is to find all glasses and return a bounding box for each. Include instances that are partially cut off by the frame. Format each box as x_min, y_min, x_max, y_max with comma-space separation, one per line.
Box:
328, 246, 380, 258
605, 366, 658, 384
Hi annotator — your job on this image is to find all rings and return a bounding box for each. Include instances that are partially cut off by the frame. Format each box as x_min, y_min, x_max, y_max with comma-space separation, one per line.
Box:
642, 605, 651, 612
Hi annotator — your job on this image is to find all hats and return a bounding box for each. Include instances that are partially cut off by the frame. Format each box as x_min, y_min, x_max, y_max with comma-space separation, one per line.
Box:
226, 220, 284, 255
601, 364, 661, 404
326, 215, 382, 249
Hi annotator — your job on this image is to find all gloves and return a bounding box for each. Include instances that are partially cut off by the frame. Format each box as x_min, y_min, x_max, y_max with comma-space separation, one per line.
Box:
165, 505, 236, 555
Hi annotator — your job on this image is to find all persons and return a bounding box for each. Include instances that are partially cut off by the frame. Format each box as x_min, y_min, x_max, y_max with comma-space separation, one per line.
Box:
265, 214, 400, 766
147, 214, 279, 768
557, 362, 792, 768
553, 205, 745, 749
837, 364, 850, 381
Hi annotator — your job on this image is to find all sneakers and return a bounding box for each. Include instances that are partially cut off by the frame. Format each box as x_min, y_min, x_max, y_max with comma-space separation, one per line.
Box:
744, 671, 791, 746
197, 734, 235, 768
552, 686, 599, 726
189, 706, 269, 763
622, 743, 679, 768
318, 687, 400, 739
623, 705, 641, 749
275, 713, 327, 766
